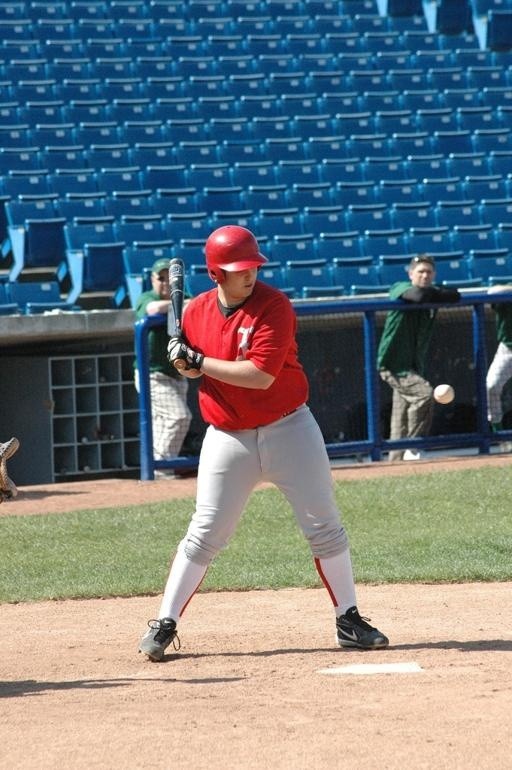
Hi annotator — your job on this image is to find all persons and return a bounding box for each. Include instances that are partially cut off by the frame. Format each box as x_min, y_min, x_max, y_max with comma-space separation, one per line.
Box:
132, 223, 391, 662
485, 283, 511, 448
131, 255, 195, 482
374, 253, 463, 464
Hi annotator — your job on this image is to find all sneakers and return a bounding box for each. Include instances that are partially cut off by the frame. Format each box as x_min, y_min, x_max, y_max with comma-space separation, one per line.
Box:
499, 439, 511, 453
333, 604, 390, 649
138, 617, 179, 663
401, 450, 429, 461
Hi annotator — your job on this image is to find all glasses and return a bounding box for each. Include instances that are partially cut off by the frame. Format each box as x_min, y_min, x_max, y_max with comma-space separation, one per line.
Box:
414, 253, 436, 270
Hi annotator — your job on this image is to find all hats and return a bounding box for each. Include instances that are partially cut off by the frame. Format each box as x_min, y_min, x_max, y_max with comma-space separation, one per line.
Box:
149, 258, 171, 274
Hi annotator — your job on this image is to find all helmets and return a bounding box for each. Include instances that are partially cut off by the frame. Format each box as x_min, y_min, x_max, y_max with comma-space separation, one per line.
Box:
205, 225, 267, 285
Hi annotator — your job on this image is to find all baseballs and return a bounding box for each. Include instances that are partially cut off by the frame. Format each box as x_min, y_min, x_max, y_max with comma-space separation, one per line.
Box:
433, 384, 456, 405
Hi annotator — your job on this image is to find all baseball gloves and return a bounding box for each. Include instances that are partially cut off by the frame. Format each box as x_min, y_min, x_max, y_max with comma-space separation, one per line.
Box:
1, 437, 19, 500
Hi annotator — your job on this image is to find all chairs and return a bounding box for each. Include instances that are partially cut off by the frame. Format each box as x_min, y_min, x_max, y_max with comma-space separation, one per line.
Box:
1, 1, 511, 312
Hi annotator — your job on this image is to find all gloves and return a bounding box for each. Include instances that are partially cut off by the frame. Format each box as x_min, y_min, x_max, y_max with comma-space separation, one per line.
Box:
168, 344, 205, 372
166, 336, 187, 356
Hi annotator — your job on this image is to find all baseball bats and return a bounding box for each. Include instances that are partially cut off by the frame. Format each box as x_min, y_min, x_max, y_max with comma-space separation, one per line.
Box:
168, 259, 187, 373
